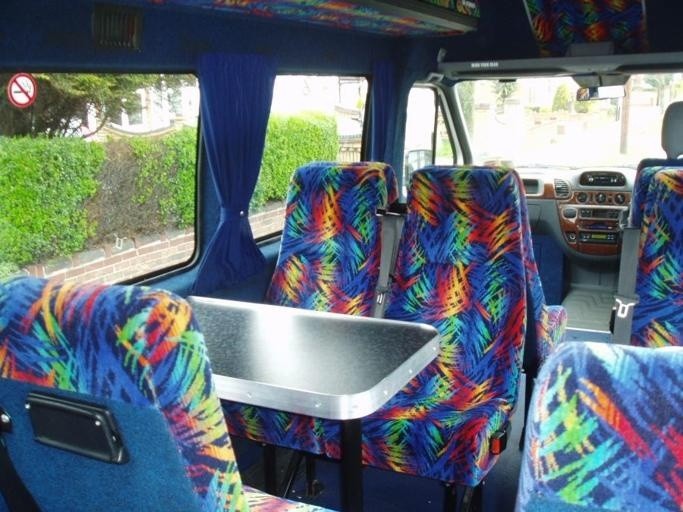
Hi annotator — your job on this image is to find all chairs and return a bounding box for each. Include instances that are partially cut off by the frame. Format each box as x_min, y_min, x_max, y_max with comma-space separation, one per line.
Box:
511, 338, 680, 508
609, 163, 683, 347
509, 166, 570, 379
0, 274, 340, 512
325, 166, 528, 508
189, 161, 393, 506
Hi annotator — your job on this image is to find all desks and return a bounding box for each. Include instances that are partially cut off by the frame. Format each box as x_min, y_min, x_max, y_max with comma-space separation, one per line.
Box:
173, 293, 444, 510
